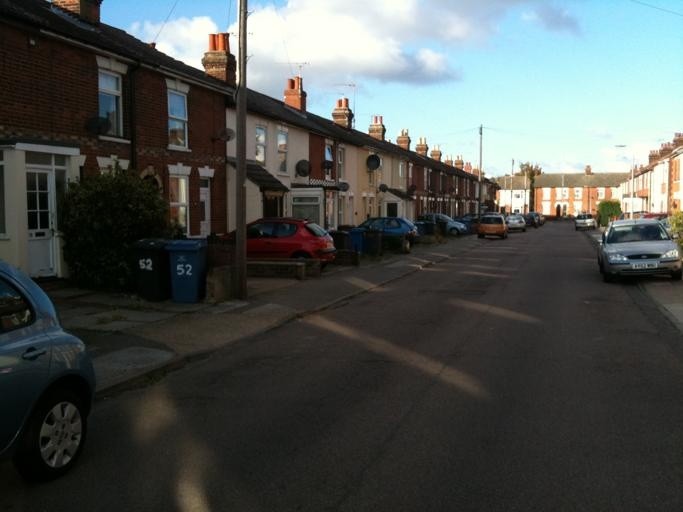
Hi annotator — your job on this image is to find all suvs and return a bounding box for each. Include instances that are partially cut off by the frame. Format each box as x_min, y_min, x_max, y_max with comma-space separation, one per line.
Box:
574, 213, 594, 231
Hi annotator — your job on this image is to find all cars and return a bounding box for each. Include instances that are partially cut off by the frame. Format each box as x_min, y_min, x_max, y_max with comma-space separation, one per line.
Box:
221, 217, 337, 270
475, 214, 508, 239
596, 219, 682, 282
344, 216, 419, 253
419, 213, 467, 237
616, 210, 678, 239
0, 259, 92, 481
455, 211, 546, 232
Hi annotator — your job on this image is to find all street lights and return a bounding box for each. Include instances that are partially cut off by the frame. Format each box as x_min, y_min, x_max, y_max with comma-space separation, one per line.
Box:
524, 166, 528, 216
509, 157, 515, 214
561, 175, 565, 217
613, 144, 634, 218
478, 123, 483, 216
504, 173, 510, 214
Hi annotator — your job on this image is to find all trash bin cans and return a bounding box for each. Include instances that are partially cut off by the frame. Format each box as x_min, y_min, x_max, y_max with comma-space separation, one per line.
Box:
164, 239, 208, 303
136, 238, 175, 302
